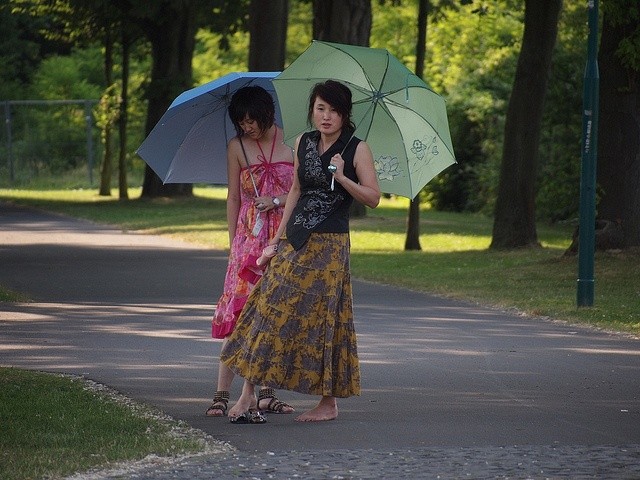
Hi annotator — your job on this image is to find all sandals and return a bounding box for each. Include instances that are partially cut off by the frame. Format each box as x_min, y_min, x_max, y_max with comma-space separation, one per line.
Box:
205, 390, 231, 416
257, 387, 294, 415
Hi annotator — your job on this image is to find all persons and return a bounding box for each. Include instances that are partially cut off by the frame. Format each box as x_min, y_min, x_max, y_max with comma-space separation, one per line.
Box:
226, 83, 361, 425
206, 85, 295, 417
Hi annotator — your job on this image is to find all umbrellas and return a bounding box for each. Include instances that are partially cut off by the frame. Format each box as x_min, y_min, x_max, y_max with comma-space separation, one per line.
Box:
269, 39, 458, 202
137, 71, 286, 211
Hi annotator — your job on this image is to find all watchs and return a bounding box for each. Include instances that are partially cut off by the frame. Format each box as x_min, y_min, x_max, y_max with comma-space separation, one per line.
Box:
272, 195, 281, 208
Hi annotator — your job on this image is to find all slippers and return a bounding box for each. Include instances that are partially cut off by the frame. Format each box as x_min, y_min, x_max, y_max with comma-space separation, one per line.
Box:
230, 413, 249, 424
248, 409, 265, 423
